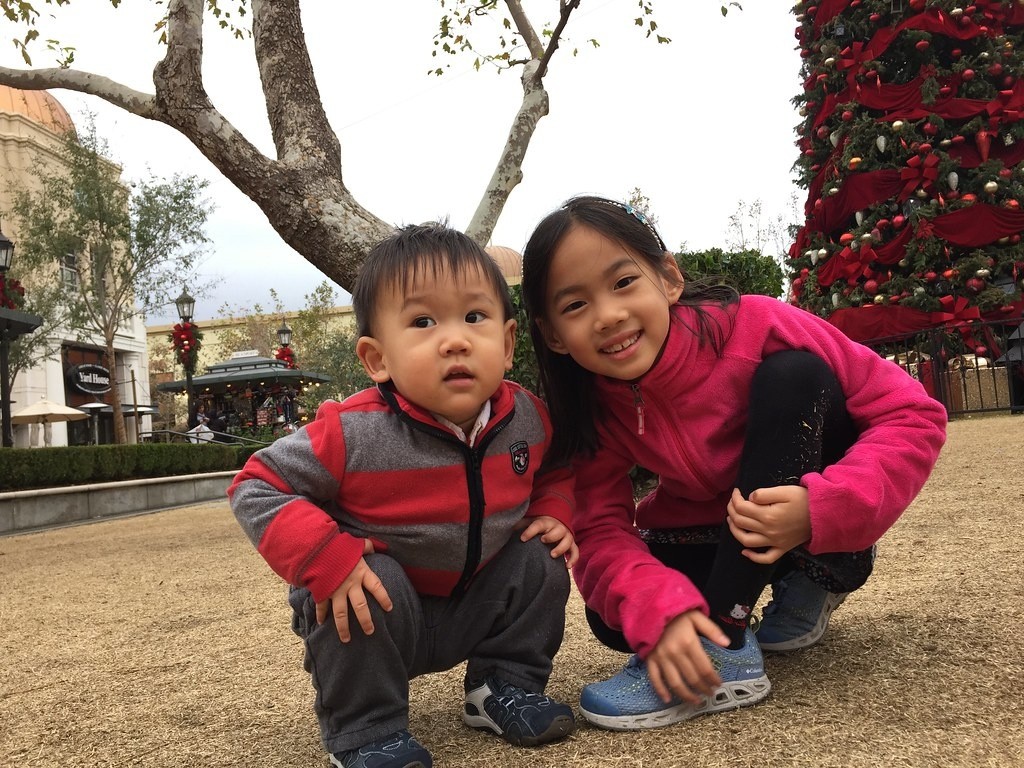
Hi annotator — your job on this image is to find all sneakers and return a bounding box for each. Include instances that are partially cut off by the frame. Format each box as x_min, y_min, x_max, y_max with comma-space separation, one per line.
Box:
463, 675, 575, 747
754, 569, 849, 650
579, 626, 771, 731
329, 729, 431, 768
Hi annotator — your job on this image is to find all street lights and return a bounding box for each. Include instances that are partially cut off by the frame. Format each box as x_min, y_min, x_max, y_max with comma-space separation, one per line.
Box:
174, 285, 198, 432
1, 226, 16, 449
277, 316, 297, 425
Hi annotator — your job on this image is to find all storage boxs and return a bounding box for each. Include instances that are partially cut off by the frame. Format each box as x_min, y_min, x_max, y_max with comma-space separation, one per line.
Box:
882, 346, 1012, 422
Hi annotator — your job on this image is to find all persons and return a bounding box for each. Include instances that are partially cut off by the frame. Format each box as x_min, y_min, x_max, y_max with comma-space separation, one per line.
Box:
518, 195, 951, 732
186, 400, 213, 429
224, 222, 583, 767
